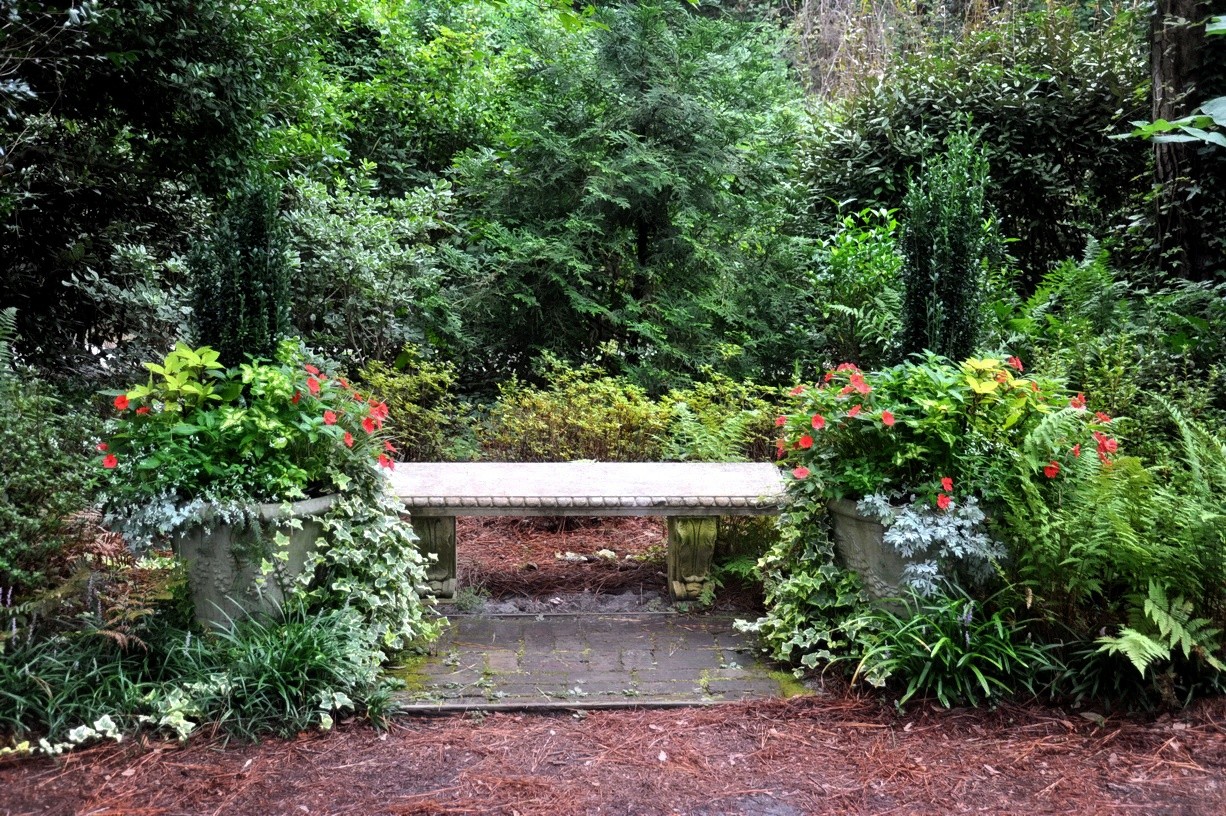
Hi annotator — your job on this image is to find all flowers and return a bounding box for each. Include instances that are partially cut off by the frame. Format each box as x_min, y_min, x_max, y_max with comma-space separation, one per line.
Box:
730, 349, 1132, 690
78, 336, 451, 742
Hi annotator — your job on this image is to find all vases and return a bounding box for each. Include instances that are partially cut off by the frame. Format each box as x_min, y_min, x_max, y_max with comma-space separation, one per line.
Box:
826, 495, 943, 622
168, 490, 346, 640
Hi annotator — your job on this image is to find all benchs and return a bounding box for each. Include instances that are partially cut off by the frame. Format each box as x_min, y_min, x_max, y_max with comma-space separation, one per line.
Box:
368, 463, 792, 616
